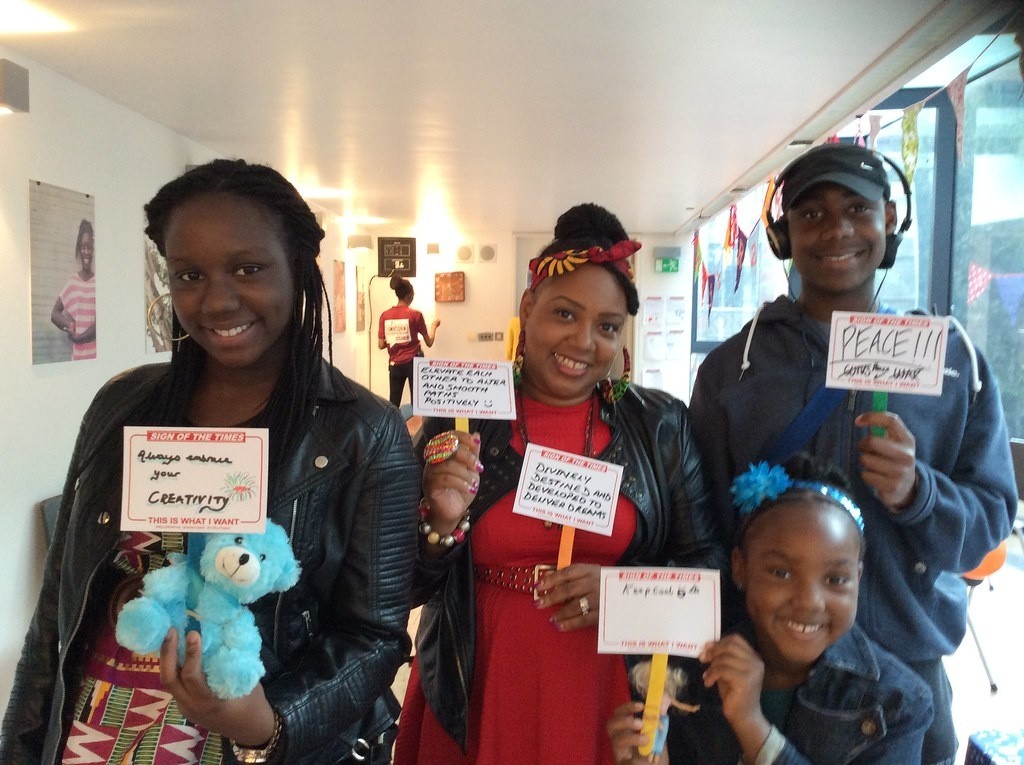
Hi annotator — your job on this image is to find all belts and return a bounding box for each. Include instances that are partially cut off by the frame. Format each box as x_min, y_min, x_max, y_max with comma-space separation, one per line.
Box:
474, 563, 558, 597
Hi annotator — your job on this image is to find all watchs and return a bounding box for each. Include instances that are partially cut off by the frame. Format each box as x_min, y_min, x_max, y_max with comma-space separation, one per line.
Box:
229, 712, 283, 763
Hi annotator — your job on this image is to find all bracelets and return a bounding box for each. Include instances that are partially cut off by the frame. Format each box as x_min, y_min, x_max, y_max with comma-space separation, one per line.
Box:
416, 493, 470, 545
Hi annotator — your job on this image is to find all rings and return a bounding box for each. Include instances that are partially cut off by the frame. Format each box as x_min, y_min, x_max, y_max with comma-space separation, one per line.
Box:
579, 597, 590, 615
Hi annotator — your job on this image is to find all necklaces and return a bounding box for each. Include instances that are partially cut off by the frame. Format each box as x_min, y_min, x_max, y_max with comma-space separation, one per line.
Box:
512, 380, 597, 529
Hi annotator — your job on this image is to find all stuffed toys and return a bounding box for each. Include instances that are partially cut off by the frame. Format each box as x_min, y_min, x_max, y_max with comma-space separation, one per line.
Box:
117, 514, 304, 698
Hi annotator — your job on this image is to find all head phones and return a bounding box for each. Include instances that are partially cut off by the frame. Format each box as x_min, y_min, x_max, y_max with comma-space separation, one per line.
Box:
761, 143, 913, 270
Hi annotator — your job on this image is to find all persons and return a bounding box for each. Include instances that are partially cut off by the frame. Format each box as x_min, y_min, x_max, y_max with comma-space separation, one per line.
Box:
1, 158, 417, 764
606, 454, 932, 763
391, 206, 720, 764
687, 139, 1018, 762
380, 273, 439, 409
51, 219, 97, 361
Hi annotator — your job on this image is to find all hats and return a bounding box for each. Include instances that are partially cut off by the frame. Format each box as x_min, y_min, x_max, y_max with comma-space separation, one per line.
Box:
780, 147, 891, 214
